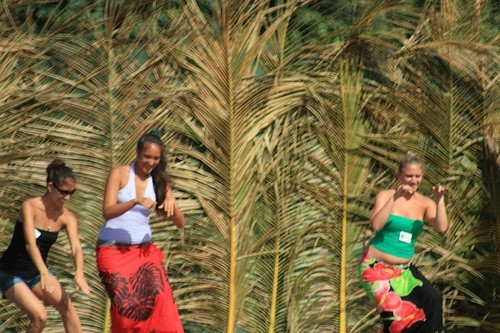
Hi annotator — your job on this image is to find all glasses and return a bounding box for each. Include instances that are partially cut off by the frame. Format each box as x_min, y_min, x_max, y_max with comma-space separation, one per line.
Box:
52, 182, 77, 195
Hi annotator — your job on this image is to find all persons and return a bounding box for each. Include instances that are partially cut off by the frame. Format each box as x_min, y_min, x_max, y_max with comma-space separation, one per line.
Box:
96, 133, 186, 333
359, 150, 448, 333
0, 159, 90, 333
